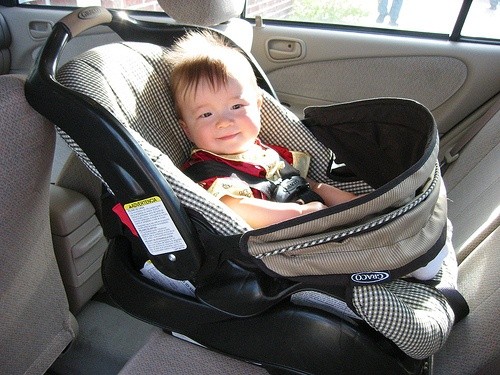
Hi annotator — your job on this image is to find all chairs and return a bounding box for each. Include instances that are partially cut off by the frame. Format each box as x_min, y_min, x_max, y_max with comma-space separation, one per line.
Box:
24, 6, 457, 374
441, 113, 500, 269
423, 227, 499, 374
164, 0, 255, 55
0, 73, 80, 372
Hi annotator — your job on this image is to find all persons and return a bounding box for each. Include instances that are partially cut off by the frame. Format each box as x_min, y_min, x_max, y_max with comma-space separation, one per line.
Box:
161, 25, 455, 282
